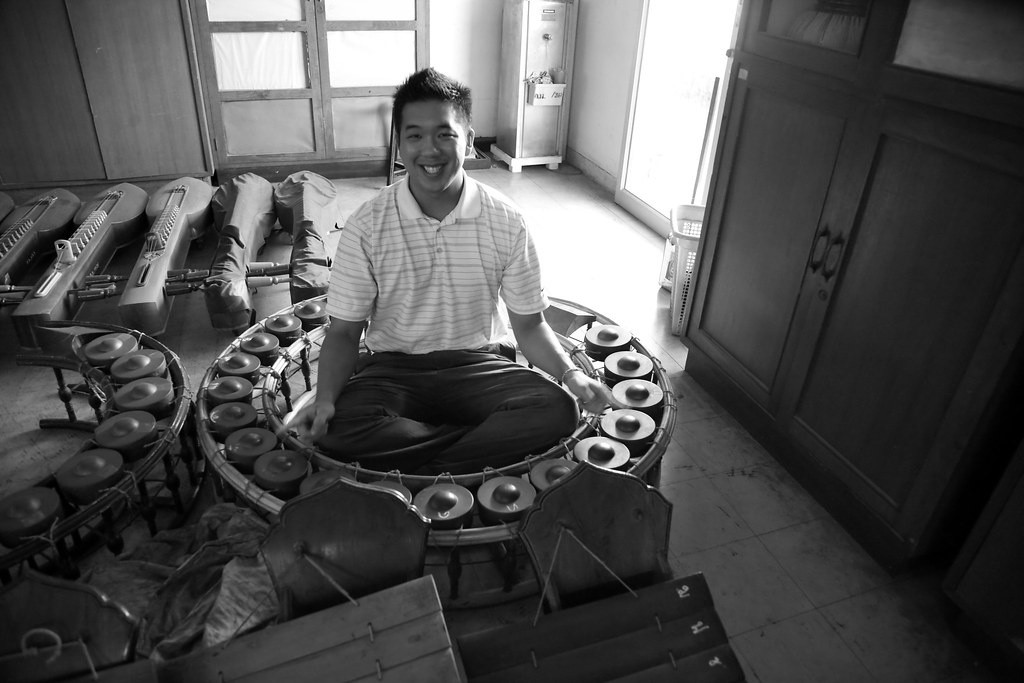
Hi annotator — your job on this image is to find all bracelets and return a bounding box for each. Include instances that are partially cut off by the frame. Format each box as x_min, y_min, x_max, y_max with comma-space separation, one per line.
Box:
562, 368, 583, 383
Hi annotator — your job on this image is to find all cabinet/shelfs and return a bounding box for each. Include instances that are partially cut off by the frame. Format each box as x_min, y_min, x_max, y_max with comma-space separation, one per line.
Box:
678, 0, 1024, 577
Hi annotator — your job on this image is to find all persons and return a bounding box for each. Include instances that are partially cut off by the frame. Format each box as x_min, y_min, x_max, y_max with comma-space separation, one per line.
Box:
275, 68, 624, 476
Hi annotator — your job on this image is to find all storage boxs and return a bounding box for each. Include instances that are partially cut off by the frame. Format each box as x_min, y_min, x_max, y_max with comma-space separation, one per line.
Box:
530, 82, 567, 106
